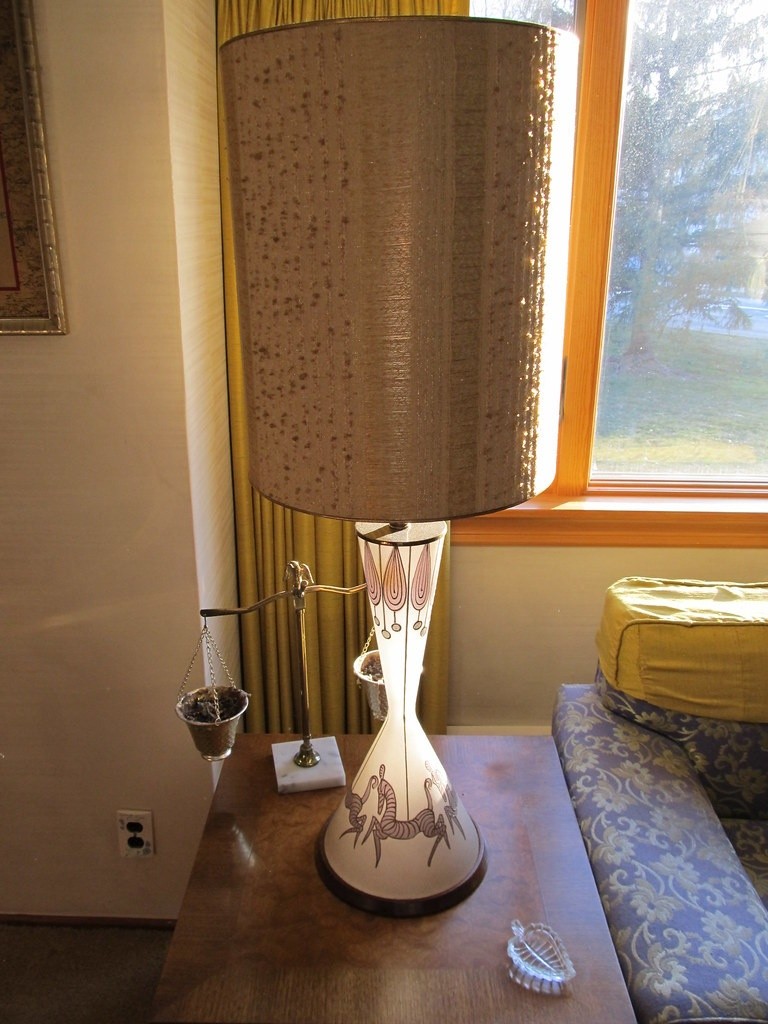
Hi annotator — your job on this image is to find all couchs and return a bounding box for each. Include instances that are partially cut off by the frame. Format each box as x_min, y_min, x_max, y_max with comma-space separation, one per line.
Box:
553, 576, 768, 1024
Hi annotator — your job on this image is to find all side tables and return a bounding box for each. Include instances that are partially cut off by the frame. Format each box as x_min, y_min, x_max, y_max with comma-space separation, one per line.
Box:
147, 733, 638, 1024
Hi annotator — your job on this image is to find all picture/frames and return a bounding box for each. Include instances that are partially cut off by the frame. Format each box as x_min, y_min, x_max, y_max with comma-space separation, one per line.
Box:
0, 0, 69, 336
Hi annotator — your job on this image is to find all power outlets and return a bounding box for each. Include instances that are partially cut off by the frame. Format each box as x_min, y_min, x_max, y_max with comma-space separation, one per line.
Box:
116, 809, 155, 858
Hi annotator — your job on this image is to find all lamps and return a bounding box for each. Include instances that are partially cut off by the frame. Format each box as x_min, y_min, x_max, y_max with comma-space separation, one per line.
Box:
218, 12, 583, 919
